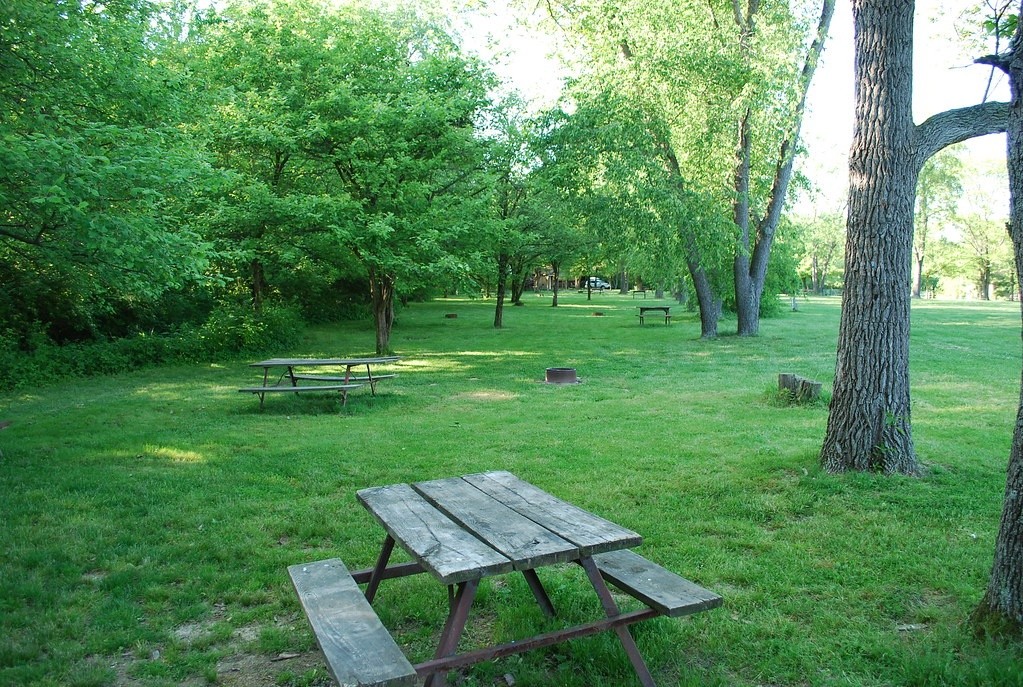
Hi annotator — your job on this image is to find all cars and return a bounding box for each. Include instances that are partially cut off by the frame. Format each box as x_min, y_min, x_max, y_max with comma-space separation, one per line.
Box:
584, 277, 610, 289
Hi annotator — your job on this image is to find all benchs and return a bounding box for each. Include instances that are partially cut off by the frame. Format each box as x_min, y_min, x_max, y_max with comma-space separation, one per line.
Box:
666, 315, 671, 318
287, 557, 417, 687
285, 374, 395, 381
239, 385, 362, 391
636, 315, 643, 317
574, 549, 724, 617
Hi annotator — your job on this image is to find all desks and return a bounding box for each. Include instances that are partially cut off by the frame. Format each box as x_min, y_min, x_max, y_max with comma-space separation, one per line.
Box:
630, 291, 645, 299
250, 357, 400, 407
636, 306, 670, 324
356, 471, 657, 687
591, 288, 603, 295
